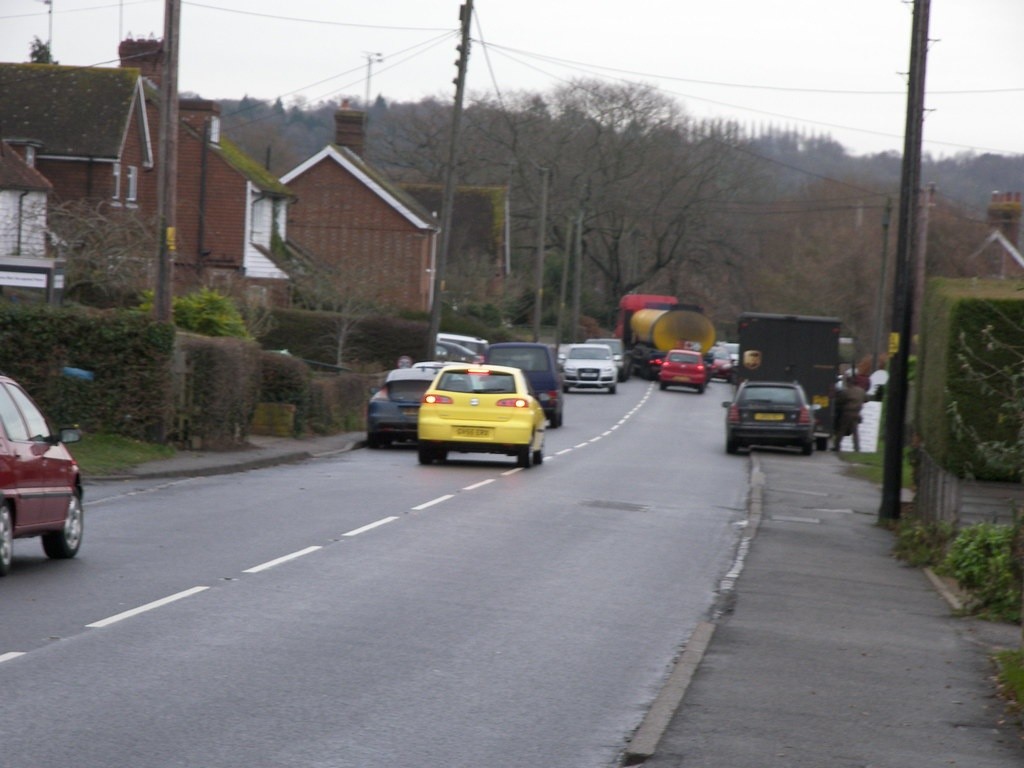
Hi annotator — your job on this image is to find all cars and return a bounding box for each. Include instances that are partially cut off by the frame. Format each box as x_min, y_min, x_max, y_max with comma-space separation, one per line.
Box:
585, 339, 630, 383
364, 369, 439, 450
708, 343, 739, 379
436, 334, 488, 355
559, 345, 623, 394
434, 341, 485, 365
722, 380, 821, 456
659, 349, 707, 392
1, 375, 85, 578
416, 364, 549, 469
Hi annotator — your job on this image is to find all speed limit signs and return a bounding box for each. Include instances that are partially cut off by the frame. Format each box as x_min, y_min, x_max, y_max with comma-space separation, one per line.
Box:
396, 356, 413, 369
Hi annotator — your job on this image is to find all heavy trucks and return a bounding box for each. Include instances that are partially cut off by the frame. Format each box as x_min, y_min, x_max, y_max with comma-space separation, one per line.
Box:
610, 292, 716, 382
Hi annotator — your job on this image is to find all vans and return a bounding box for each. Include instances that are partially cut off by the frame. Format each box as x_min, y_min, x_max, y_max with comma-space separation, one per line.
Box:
484, 342, 564, 430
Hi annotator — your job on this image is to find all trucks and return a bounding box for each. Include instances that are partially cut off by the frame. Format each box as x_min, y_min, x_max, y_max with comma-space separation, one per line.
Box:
739, 314, 842, 451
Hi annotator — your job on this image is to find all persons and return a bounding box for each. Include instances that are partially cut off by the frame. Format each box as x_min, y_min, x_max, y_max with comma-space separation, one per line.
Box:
829, 375, 867, 453
